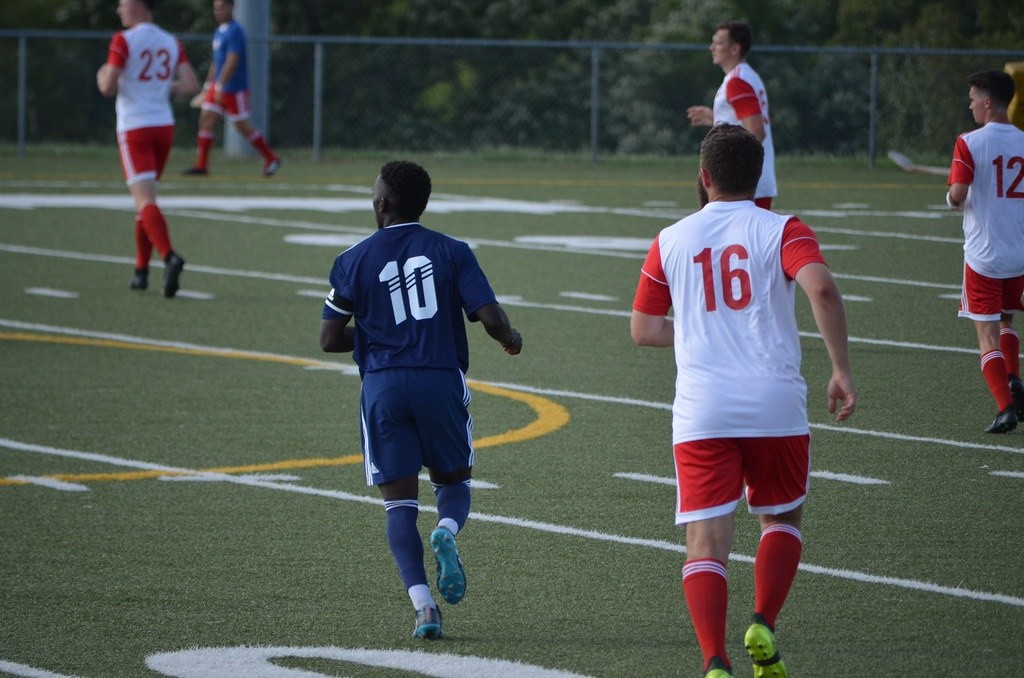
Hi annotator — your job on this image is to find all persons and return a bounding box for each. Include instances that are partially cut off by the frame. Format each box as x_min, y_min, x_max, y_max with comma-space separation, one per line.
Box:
944, 70, 1024, 433
189, 0, 284, 178
96, 0, 201, 299
686, 23, 778, 213
629, 123, 857, 678
318, 163, 523, 642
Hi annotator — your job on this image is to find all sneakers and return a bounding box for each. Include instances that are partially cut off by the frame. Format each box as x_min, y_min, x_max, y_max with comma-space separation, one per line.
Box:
131, 267, 149, 289
412, 604, 443, 641
702, 656, 734, 678
429, 525, 467, 604
984, 408, 1018, 435
164, 252, 185, 299
744, 612, 788, 678
1007, 380, 1024, 424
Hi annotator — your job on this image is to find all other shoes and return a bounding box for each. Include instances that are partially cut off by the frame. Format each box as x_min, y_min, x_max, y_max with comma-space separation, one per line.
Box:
262, 157, 284, 177
182, 167, 207, 176
